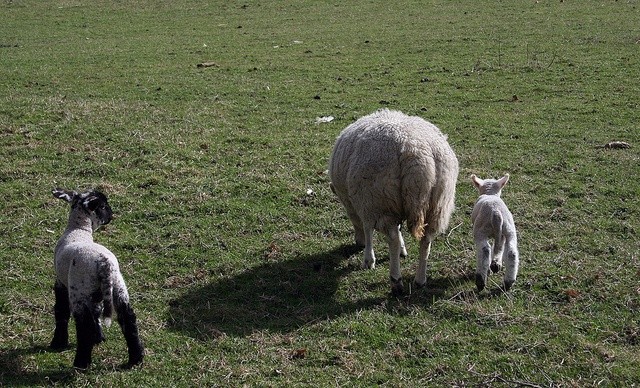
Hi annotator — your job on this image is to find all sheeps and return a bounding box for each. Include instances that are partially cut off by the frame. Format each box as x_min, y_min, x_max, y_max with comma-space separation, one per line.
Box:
470, 172, 519, 296
49, 186, 147, 373
327, 106, 461, 298
